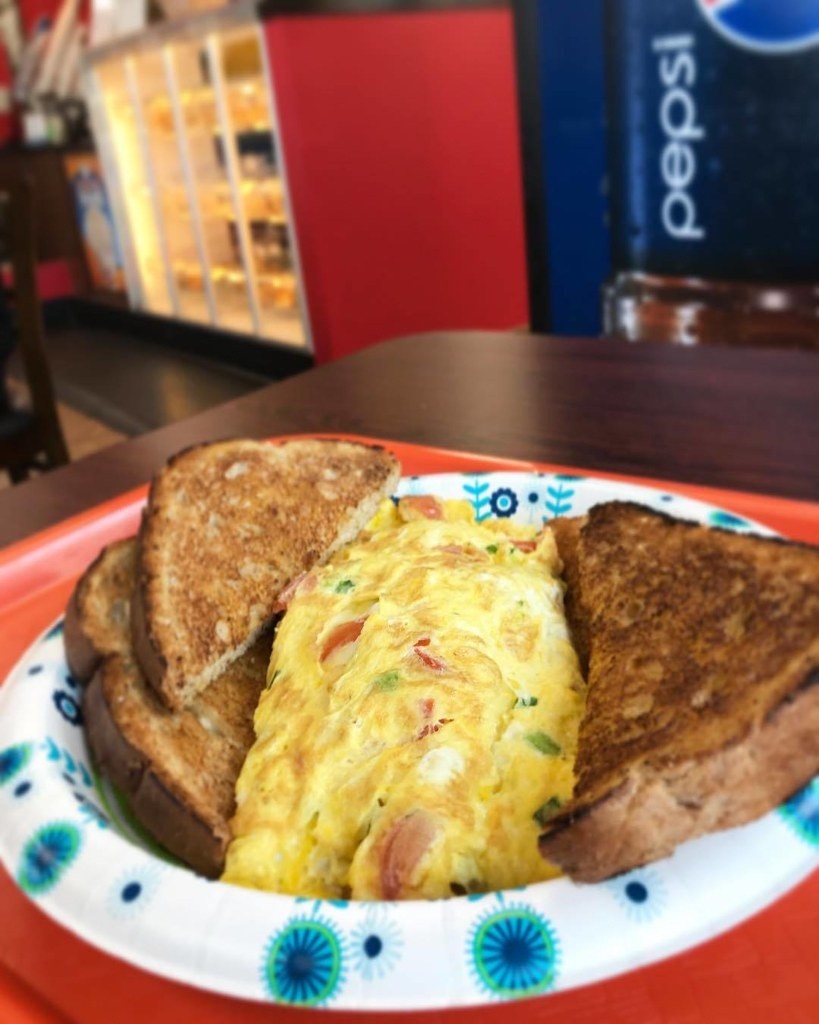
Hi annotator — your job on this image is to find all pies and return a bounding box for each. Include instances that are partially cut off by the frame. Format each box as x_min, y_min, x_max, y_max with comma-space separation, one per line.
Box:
219, 494, 589, 899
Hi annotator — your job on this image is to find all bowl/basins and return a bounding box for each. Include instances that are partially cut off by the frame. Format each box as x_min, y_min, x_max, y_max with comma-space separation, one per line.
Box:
0, 470, 817, 1015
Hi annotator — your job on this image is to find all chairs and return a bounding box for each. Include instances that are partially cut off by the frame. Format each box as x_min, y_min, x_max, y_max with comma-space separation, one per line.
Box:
0, 229, 70, 480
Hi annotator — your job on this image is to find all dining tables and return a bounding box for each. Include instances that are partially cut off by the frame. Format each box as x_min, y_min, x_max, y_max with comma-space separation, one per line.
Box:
3, 328, 819, 1022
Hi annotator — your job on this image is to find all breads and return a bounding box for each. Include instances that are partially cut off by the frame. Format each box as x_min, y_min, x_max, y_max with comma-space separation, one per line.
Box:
538, 495, 818, 883
64, 435, 399, 878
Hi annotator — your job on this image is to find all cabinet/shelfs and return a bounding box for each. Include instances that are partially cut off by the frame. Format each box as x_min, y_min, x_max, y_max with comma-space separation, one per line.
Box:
73, 0, 534, 359
